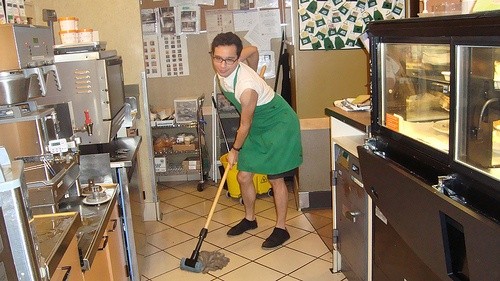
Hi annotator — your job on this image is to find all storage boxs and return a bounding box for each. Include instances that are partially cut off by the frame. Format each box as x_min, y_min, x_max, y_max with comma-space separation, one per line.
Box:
173, 96, 204, 122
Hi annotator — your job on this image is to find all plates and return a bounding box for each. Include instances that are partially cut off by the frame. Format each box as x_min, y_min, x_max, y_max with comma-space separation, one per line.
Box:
82, 195, 111, 205
81, 187, 106, 196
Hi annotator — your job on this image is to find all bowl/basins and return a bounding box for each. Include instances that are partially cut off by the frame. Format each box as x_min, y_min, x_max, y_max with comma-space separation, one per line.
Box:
59, 16, 94, 44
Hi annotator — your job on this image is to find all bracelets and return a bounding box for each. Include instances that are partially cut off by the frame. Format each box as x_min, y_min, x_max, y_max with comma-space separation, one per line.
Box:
232, 146, 242, 151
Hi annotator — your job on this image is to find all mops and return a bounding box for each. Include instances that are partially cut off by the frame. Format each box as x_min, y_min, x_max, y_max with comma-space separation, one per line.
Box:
178, 161, 230, 274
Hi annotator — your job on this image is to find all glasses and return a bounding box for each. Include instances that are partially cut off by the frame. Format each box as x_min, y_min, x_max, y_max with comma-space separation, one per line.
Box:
211, 55, 240, 65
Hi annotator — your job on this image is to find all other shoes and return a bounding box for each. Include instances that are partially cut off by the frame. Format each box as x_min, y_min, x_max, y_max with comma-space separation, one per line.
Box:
227, 218, 258, 236
261, 226, 290, 249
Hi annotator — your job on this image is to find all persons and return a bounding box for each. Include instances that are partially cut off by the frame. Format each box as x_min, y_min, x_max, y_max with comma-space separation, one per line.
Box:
209, 32, 303, 249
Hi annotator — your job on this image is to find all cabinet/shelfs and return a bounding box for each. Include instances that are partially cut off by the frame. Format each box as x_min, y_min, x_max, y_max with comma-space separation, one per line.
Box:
336, 158, 372, 281
52, 234, 83, 281
211, 74, 240, 182
152, 119, 206, 189
88, 201, 129, 281
